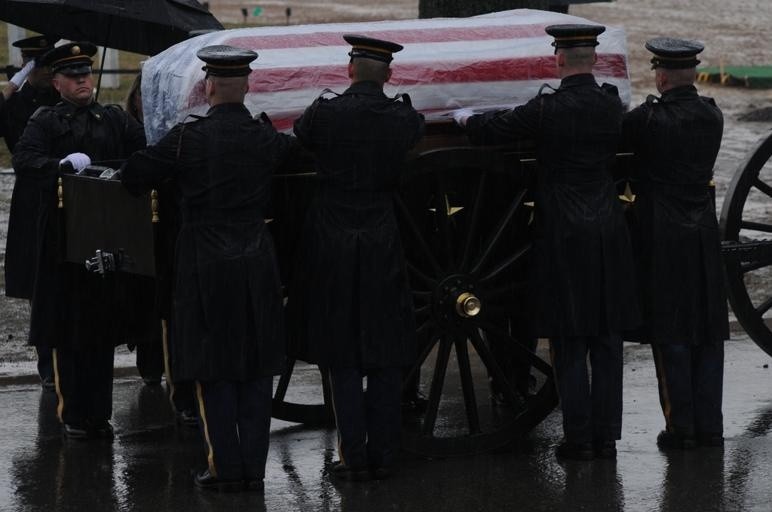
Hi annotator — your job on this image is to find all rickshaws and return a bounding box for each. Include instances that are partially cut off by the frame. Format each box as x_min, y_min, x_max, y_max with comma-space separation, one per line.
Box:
49, 99, 664, 447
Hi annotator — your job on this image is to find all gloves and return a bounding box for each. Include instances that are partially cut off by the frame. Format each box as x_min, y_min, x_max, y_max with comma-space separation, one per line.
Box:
59, 154, 91, 173
452, 105, 474, 123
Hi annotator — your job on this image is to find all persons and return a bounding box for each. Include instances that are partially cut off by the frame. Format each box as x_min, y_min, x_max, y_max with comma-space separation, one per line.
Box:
451, 25, 730, 462
1, 35, 426, 489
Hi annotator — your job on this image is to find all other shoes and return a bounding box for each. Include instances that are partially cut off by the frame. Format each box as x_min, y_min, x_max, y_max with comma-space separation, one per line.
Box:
329, 459, 373, 484
658, 429, 703, 450
142, 379, 266, 496
63, 423, 91, 439
596, 438, 617, 458
89, 416, 114, 438
556, 442, 597, 463
42, 378, 57, 392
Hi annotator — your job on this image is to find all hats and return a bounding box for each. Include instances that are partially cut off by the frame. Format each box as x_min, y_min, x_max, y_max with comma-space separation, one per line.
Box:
342, 33, 404, 64
13, 35, 59, 61
197, 46, 258, 76
39, 41, 98, 76
544, 23, 607, 48
645, 38, 703, 71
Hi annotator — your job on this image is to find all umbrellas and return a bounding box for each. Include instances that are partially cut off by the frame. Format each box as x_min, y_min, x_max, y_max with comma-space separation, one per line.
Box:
0, 0, 223, 100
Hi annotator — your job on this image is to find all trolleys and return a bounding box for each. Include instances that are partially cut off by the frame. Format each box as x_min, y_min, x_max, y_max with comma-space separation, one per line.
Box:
719, 108, 771, 369
